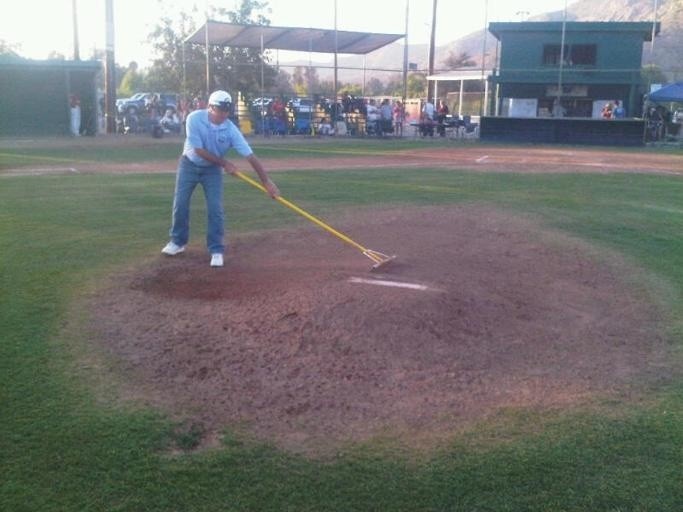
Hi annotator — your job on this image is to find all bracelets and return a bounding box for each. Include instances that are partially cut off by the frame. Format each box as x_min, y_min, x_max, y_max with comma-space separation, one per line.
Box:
222, 160, 225, 167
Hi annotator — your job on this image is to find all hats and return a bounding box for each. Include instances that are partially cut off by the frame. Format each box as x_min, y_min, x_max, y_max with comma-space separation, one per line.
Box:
208, 90, 231, 107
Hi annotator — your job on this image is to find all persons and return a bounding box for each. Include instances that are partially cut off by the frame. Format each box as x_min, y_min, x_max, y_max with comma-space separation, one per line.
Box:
646, 107, 683, 146
600, 102, 613, 119
160, 90, 280, 268
612, 100, 625, 120
552, 99, 567, 117
69, 89, 82, 137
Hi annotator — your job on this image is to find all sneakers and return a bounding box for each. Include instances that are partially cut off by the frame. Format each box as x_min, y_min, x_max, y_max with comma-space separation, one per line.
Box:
209, 253, 223, 267
161, 241, 184, 255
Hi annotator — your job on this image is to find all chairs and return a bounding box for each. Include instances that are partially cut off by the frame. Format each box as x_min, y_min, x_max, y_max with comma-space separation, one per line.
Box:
249, 97, 479, 143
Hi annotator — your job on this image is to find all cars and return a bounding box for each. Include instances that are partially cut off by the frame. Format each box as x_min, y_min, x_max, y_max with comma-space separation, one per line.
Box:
116, 87, 383, 134
